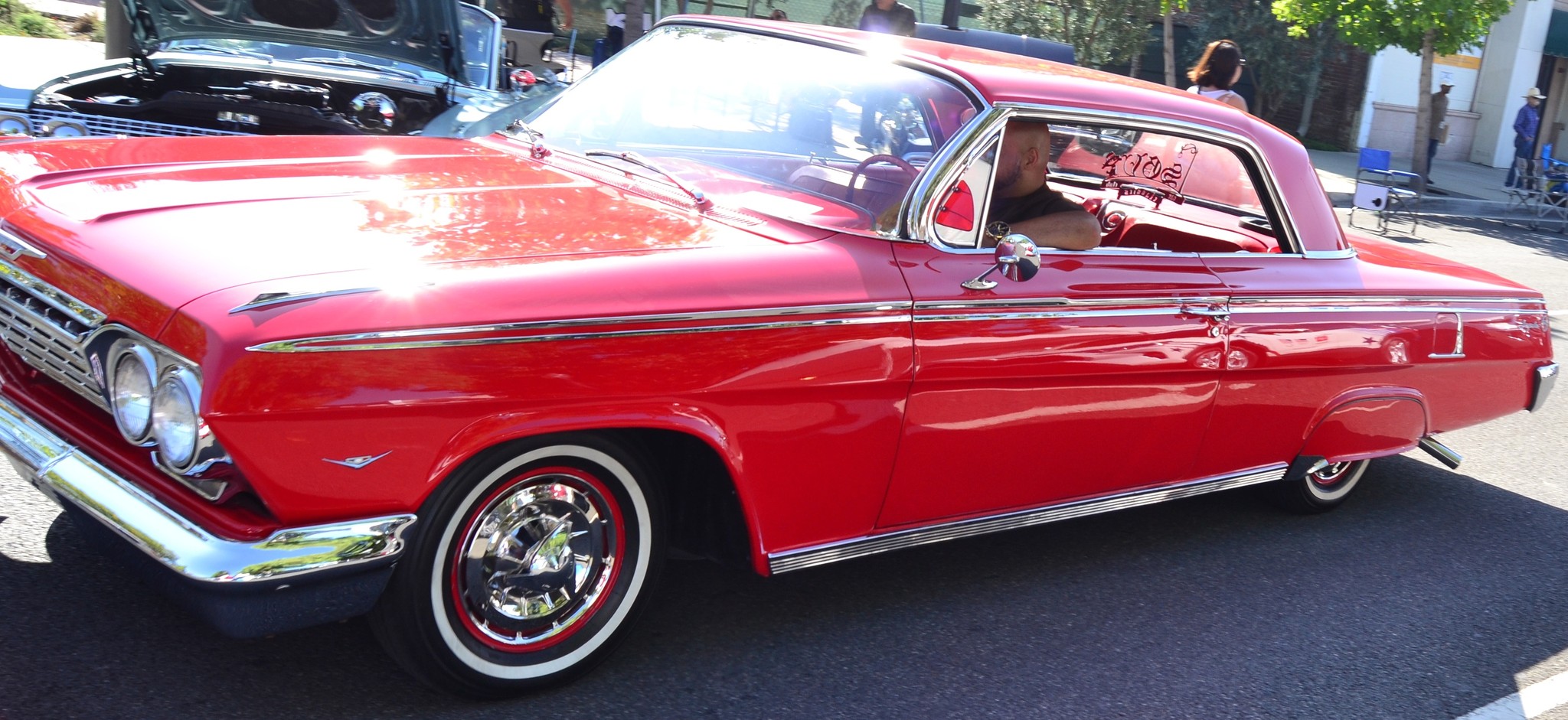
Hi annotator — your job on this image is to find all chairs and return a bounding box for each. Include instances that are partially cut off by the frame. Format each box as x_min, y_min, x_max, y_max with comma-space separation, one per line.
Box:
786, 163, 912, 224
1503, 157, 1567, 234
1533, 142, 1568, 219
1348, 146, 1424, 236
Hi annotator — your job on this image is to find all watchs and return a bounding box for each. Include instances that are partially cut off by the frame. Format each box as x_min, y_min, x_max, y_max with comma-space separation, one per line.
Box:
984, 220, 1012, 245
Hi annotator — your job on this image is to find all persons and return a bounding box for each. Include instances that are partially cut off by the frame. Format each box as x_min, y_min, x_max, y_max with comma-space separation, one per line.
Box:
1186, 39, 1249, 113
854, 0, 918, 149
1500, 87, 1547, 195
770, 9, 787, 21
977, 119, 1102, 251
499, 0, 574, 49
1426, 79, 1455, 184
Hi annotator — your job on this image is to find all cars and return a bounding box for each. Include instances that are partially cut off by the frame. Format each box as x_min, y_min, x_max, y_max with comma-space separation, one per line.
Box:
23, 0, 572, 146
0, 12, 1556, 706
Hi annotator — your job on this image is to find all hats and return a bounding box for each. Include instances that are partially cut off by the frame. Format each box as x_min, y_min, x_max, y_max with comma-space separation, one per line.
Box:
1440, 78, 1455, 87
1520, 87, 1547, 99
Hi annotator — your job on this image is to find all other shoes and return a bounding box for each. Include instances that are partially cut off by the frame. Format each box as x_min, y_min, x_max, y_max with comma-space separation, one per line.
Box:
1426, 177, 1434, 184
1501, 186, 1517, 195
